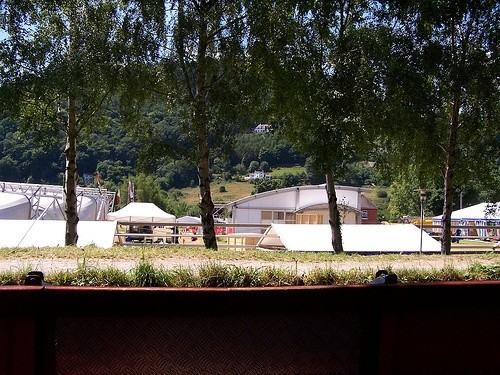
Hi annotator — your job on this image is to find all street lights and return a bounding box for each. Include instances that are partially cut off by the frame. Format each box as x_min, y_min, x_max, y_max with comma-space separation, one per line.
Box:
419, 191, 427, 254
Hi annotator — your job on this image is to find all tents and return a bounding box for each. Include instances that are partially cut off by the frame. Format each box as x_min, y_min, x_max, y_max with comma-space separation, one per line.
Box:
175, 216, 202, 225
106, 202, 176, 243
431, 202, 500, 239
